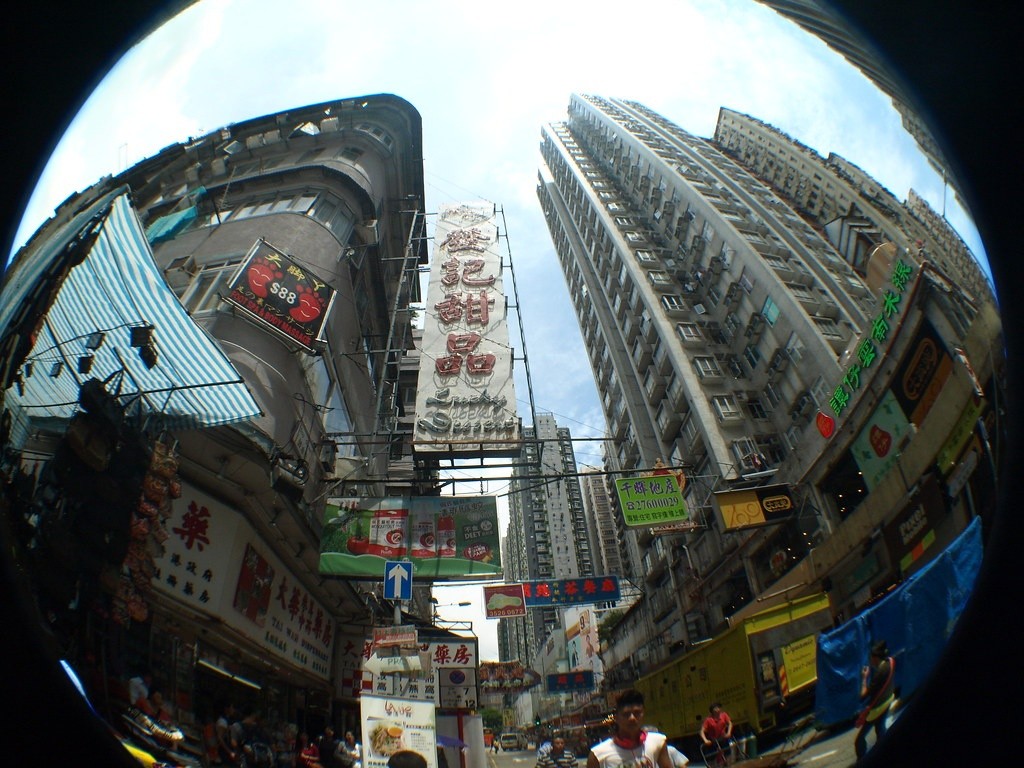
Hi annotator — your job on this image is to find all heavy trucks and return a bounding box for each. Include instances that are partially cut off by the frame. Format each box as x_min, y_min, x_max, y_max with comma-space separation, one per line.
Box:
634, 580, 835, 741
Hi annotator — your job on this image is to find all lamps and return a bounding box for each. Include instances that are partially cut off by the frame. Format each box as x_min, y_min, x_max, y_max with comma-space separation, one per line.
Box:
15, 322, 157, 397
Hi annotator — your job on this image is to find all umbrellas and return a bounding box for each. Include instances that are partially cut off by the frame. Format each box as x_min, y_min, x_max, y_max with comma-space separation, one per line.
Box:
436, 734, 468, 751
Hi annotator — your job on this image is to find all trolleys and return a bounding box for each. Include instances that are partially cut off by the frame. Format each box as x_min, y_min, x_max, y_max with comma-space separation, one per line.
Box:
699, 735, 787, 768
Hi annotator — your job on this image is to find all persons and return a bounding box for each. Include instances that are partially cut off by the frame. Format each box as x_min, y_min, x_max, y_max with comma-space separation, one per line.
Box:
533, 690, 689, 768
699, 701, 734, 768
489, 736, 500, 755
568, 636, 598, 682
128, 666, 363, 768
852, 640, 897, 764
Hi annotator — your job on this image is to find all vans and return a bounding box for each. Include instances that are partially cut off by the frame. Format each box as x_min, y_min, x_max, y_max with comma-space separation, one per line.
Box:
500, 733, 528, 750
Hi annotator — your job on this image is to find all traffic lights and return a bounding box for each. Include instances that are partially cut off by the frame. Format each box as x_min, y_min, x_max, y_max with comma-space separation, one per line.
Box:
536, 717, 541, 725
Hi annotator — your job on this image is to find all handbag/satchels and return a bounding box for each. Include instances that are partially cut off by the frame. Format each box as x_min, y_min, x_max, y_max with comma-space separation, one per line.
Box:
856, 706, 870, 727
